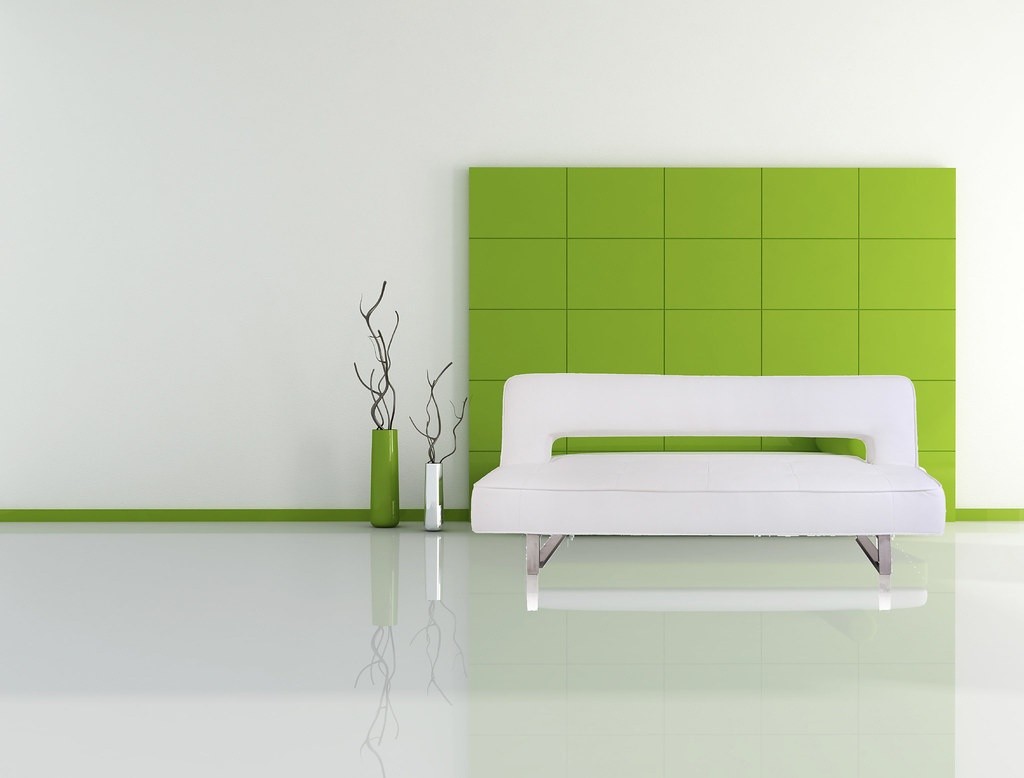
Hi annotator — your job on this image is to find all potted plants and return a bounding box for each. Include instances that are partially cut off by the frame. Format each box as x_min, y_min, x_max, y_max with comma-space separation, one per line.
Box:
409, 532, 469, 707
353, 280, 400, 528
409, 360, 469, 531
353, 527, 400, 778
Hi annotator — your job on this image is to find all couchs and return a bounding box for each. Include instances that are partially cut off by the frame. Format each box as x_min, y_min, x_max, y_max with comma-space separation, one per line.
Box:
470, 373, 947, 576
517, 575, 930, 612
